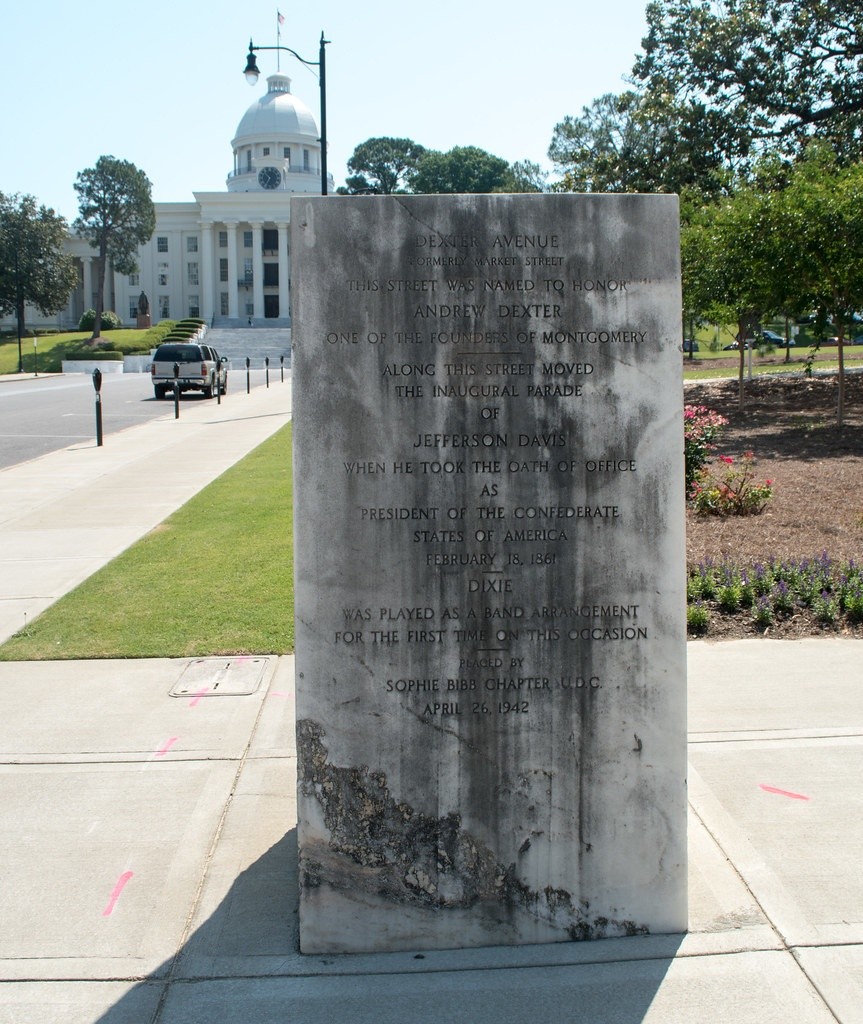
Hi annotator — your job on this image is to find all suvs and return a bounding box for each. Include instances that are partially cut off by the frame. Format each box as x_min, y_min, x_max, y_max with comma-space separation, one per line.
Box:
151, 343, 228, 399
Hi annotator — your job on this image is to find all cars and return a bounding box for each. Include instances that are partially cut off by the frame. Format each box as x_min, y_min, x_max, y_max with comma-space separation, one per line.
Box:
763, 331, 795, 348
723, 344, 733, 350
796, 310, 819, 323
809, 337, 853, 347
684, 339, 699, 352
850, 335, 863, 345
732, 330, 763, 349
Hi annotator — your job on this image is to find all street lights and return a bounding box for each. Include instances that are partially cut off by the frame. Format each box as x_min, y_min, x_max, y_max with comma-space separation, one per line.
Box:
243, 40, 331, 195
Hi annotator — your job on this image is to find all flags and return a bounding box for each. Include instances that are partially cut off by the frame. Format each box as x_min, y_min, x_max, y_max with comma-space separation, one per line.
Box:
277, 12, 285, 24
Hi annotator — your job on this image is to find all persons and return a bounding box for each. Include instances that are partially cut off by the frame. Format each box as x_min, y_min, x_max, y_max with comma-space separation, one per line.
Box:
138, 291, 149, 314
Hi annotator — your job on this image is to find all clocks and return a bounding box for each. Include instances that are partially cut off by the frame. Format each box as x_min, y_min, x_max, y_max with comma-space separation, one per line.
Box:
258, 167, 281, 190
